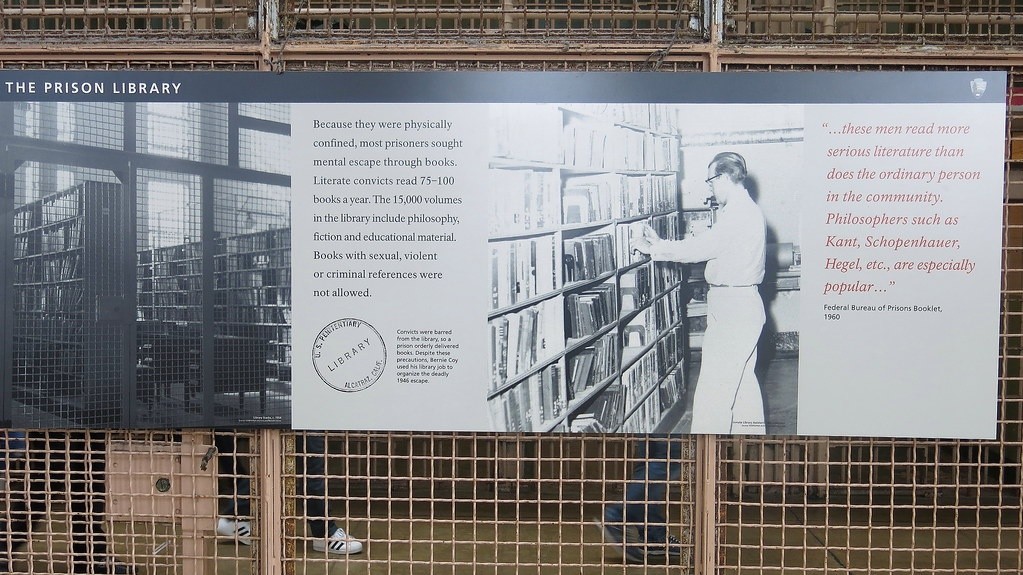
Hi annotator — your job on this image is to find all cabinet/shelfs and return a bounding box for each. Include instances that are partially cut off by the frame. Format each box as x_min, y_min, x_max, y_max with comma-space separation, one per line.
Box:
10, 181, 137, 423
481, 106, 689, 437
136, 227, 294, 393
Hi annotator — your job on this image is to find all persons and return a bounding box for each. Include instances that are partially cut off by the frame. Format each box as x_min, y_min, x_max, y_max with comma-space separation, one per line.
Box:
628, 151, 767, 436
593, 434, 680, 561
214, 429, 362, 554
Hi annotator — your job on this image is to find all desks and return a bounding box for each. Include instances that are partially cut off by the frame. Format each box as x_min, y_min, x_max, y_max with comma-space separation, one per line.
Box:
147, 335, 269, 414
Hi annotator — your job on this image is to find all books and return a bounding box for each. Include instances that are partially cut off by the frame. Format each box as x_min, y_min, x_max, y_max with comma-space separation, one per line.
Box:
485, 105, 685, 434
14, 188, 292, 395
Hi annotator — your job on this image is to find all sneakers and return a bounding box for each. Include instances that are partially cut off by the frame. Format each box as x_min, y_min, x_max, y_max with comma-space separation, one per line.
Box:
215, 517, 252, 546
313, 526, 363, 555
592, 512, 646, 564
638, 533, 682, 555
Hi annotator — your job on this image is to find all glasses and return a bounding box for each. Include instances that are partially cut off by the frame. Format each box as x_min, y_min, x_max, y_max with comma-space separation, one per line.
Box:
705, 174, 723, 186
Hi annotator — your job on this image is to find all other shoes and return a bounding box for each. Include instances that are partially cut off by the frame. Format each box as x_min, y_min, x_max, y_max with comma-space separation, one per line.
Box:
76, 554, 137, 575
